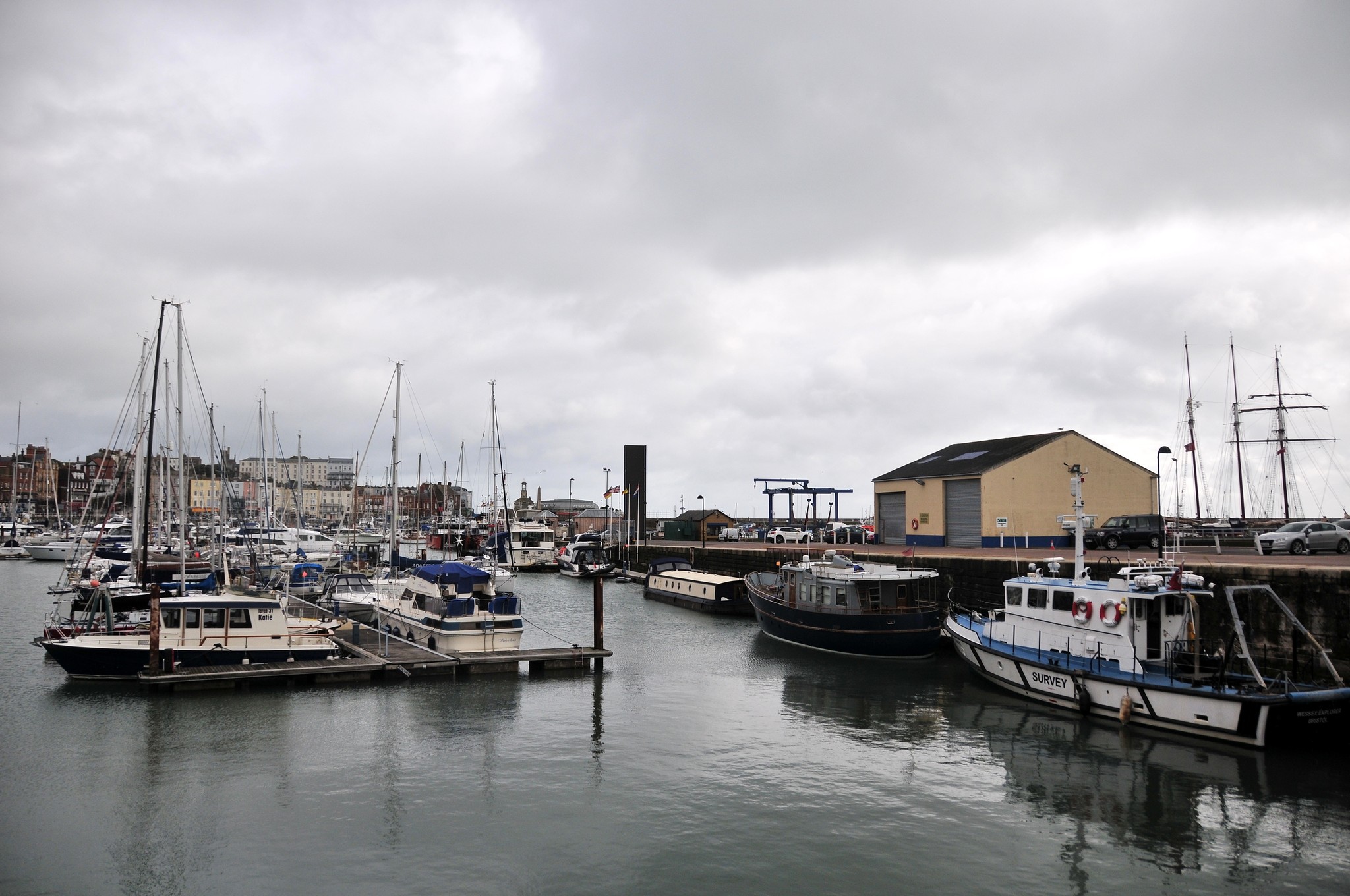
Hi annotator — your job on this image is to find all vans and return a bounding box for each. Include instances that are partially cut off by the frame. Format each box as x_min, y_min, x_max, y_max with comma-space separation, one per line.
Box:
718, 528, 740, 542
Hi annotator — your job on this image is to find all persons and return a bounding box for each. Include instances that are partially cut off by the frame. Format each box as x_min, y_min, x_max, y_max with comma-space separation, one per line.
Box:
229, 565, 242, 586
464, 524, 470, 535
420, 518, 438, 536
748, 524, 761, 538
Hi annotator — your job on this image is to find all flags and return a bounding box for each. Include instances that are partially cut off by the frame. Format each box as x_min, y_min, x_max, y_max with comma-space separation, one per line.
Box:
604, 486, 612, 499
903, 545, 914, 557
1277, 445, 1286, 454
632, 484, 638, 497
1166, 561, 1184, 591
1184, 441, 1195, 452
478, 501, 494, 509
622, 484, 629, 495
609, 485, 620, 493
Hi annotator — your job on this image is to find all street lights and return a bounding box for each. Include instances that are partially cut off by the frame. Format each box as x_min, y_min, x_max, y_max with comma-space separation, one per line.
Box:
603, 467, 611, 530
572, 508, 578, 537
697, 495, 705, 548
568, 478, 575, 536
1157, 446, 1172, 562
1172, 457, 1179, 535
603, 505, 609, 540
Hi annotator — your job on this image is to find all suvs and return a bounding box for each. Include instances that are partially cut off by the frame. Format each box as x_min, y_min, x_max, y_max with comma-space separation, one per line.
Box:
1083, 513, 1164, 550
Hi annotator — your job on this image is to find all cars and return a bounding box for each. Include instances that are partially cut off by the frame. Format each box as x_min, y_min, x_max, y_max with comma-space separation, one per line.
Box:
823, 522, 874, 544
1255, 521, 1350, 555
1325, 518, 1350, 531
574, 529, 628, 540
766, 527, 814, 543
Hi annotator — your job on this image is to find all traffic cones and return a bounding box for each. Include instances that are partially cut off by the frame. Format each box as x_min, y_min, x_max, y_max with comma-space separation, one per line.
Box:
1049, 540, 1056, 550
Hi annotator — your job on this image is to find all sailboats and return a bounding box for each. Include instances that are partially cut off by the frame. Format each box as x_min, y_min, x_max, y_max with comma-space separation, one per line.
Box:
0, 301, 525, 677
1164, 331, 1350, 538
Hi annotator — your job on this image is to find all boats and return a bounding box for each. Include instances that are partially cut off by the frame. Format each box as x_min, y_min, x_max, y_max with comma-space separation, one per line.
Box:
945, 462, 1350, 754
554, 533, 616, 579
744, 511, 949, 671
614, 577, 630, 583
504, 509, 554, 565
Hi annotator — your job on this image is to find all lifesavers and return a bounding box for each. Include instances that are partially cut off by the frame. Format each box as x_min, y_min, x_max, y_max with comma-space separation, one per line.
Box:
911, 519, 918, 530
1072, 596, 1093, 624
1100, 598, 1121, 627
